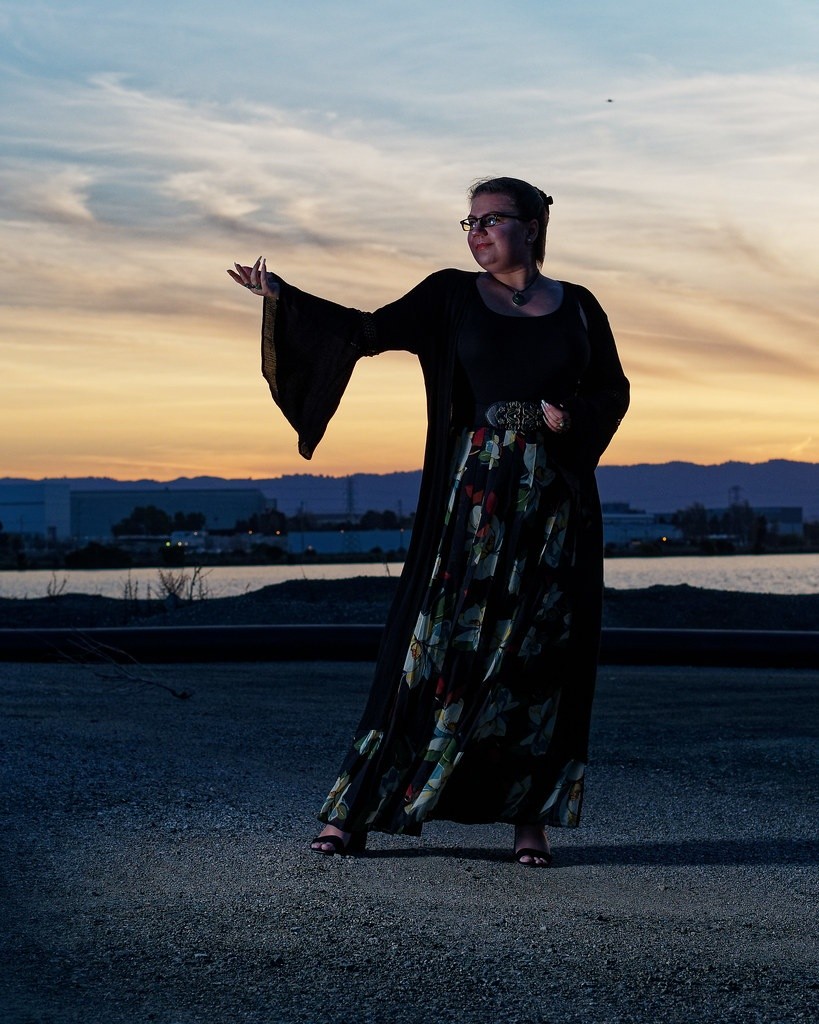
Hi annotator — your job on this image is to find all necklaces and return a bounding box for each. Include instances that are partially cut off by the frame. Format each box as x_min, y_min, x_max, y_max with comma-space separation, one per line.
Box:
486, 269, 540, 306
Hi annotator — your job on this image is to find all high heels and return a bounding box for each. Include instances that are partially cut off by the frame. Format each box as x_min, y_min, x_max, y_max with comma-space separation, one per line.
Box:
310, 831, 367, 855
515, 828, 552, 866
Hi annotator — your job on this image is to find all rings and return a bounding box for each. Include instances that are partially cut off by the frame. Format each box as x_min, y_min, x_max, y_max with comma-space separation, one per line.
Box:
244, 282, 262, 291
558, 419, 564, 427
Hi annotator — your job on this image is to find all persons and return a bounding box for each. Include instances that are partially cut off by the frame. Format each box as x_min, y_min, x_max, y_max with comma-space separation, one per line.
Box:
227, 177, 630, 867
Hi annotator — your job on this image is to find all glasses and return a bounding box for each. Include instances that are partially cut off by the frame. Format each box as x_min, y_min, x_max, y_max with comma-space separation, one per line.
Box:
460, 213, 521, 231
